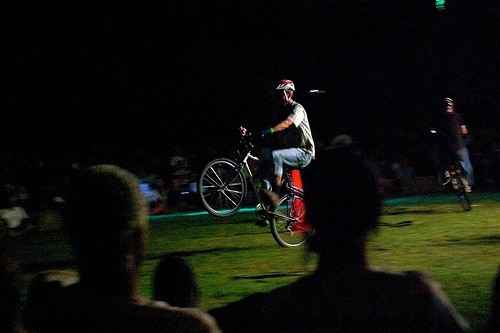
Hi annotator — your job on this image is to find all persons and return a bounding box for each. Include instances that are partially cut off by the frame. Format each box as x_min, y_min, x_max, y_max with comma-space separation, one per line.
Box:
0, 150, 218, 333
428, 98, 474, 193
235, 80, 315, 208
209, 137, 470, 333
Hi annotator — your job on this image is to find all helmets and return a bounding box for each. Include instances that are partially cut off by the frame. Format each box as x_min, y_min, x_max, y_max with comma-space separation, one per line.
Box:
276, 80, 295, 91
441, 97, 453, 106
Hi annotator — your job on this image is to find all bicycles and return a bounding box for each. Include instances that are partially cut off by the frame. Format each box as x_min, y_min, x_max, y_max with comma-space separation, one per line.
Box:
440, 157, 471, 211
198, 126, 314, 247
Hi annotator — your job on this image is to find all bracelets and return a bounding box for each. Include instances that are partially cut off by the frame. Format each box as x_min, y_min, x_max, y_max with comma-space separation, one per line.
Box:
263, 126, 274, 138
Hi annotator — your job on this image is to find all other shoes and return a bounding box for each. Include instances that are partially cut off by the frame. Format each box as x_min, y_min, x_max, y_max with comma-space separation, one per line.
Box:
262, 188, 279, 207
